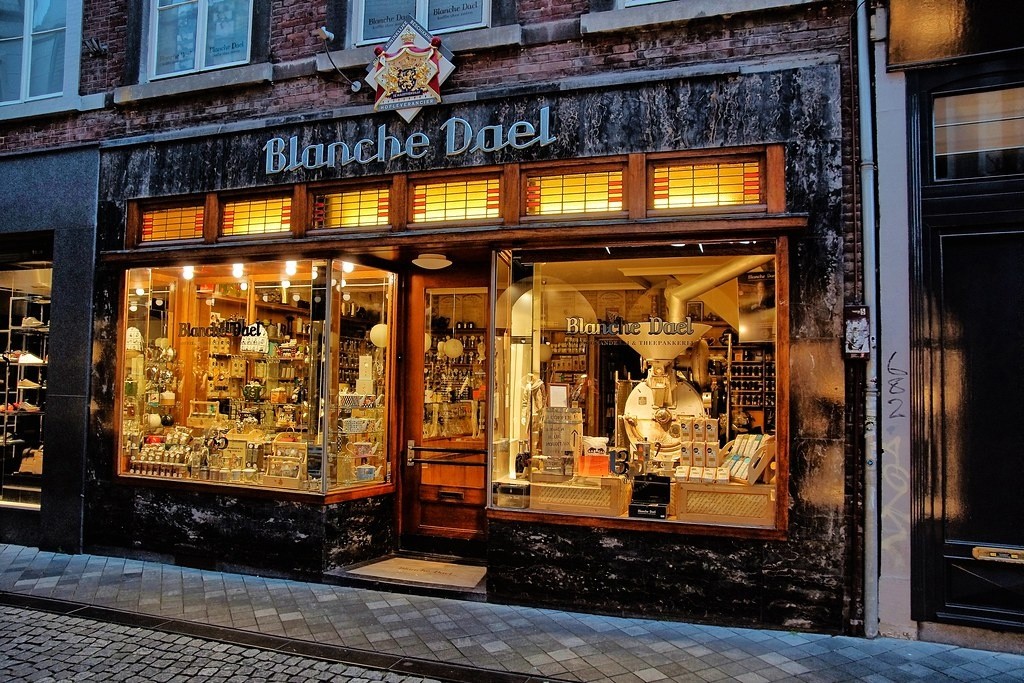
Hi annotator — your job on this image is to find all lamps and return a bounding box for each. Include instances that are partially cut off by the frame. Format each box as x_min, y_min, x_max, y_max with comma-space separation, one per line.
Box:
318, 26, 362, 93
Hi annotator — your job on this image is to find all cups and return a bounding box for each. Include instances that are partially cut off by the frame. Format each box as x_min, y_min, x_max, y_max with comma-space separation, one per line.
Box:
200, 466, 209, 479
242, 469, 256, 482
219, 468, 230, 481
210, 466, 220, 480
231, 469, 242, 481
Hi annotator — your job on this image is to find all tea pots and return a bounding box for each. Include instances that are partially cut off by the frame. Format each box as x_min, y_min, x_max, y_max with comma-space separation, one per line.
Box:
338, 416, 368, 432
340, 392, 367, 408
239, 385, 267, 402
361, 418, 383, 432
352, 464, 383, 481
346, 442, 379, 456
234, 409, 266, 425
362, 394, 384, 408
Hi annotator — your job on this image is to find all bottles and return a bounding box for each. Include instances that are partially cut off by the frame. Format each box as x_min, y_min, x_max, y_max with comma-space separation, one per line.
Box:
297, 316, 302, 333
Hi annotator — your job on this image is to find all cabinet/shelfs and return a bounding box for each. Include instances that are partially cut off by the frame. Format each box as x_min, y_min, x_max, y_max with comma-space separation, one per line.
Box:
684, 343, 776, 442
341, 335, 385, 399
424, 329, 487, 400
198, 295, 313, 421
0, 288, 51, 460
543, 325, 588, 439
